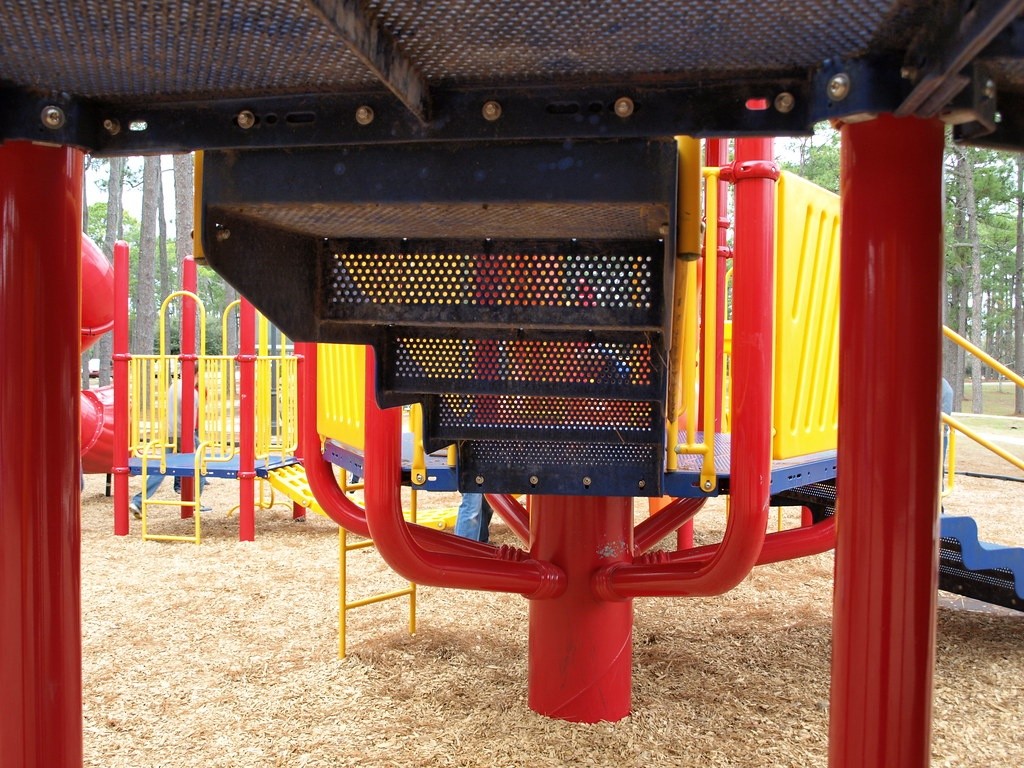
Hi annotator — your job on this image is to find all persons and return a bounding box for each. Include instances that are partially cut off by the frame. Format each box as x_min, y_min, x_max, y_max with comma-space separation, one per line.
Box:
941, 376, 953, 513
453, 492, 494, 544
130, 364, 211, 520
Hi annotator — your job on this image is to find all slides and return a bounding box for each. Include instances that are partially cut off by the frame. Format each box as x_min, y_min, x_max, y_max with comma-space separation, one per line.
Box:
79, 232, 132, 475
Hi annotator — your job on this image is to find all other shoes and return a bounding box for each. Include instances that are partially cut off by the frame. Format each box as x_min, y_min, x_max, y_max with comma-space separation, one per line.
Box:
193, 504, 213, 514
129, 503, 149, 519
